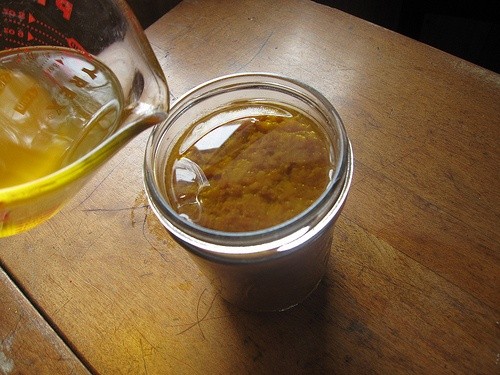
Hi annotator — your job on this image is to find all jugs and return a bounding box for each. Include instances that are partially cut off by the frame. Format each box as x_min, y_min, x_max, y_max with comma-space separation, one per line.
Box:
0, 0, 171, 239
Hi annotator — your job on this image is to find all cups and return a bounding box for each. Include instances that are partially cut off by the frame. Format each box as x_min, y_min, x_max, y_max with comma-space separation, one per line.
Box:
143, 72, 353, 312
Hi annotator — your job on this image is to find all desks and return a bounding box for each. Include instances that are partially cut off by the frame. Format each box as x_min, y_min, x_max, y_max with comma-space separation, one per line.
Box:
0, 0, 500, 375
